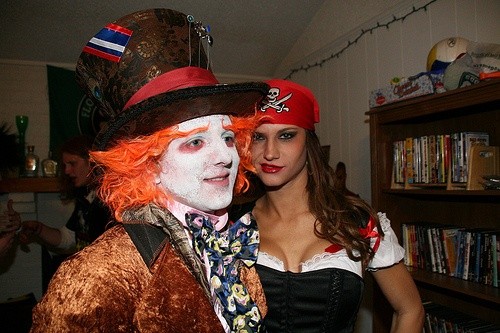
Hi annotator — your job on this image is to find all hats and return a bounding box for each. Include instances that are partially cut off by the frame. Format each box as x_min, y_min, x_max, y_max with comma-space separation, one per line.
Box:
76, 8, 271, 157
252, 79, 320, 130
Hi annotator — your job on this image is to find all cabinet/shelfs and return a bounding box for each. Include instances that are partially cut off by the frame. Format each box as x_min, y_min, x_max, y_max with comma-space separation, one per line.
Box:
365, 76, 500, 333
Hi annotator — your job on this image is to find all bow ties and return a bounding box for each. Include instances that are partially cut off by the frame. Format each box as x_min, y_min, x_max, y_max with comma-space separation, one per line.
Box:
184, 212, 267, 333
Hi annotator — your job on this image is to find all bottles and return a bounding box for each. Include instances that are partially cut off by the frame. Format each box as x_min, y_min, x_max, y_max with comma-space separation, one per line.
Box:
41, 152, 56, 177
24, 146, 39, 177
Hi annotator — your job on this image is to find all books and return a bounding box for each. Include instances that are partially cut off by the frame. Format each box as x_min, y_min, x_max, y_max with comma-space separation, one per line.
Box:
420, 299, 500, 332
390, 131, 500, 190
400, 220, 500, 287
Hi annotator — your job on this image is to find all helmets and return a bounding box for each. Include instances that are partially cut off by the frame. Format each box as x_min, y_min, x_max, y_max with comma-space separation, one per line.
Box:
427, 36, 471, 72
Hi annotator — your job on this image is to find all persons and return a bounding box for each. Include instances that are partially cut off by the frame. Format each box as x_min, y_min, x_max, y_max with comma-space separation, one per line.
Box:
32, 8, 272, 333
249, 78, 423, 333
0, 132, 120, 265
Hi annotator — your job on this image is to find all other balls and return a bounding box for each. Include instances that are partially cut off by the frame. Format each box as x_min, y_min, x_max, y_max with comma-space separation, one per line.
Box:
426, 37, 471, 72
